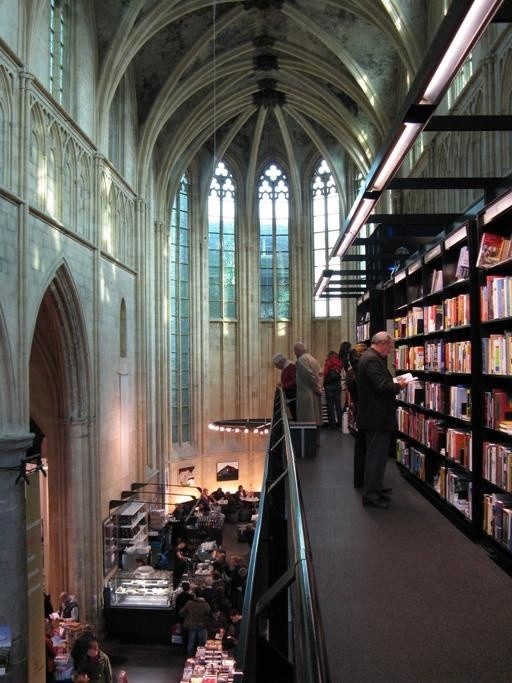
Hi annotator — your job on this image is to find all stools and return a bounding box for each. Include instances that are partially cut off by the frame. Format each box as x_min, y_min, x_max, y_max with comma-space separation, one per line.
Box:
207, 495, 260, 543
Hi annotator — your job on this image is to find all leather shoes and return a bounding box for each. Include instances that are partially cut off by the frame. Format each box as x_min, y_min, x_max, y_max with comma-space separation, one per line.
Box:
361, 487, 393, 511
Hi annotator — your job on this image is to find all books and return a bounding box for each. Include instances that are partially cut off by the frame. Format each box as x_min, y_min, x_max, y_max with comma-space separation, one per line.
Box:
180, 639, 243, 683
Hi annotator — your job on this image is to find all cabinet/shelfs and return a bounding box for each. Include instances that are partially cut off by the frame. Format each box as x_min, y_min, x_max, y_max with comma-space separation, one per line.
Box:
381, 279, 392, 375
101, 497, 222, 645
424, 217, 475, 546
355, 289, 382, 348
476, 187, 512, 577
392, 254, 424, 493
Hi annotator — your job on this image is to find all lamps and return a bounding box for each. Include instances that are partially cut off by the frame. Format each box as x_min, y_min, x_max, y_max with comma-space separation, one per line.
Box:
206, 416, 273, 436
108, 480, 206, 518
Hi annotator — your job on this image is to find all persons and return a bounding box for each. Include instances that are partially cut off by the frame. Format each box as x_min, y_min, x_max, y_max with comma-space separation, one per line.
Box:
56, 592, 78, 622
78, 641, 113, 683
271, 330, 406, 509
175, 484, 260, 657
46, 623, 60, 682
72, 626, 98, 668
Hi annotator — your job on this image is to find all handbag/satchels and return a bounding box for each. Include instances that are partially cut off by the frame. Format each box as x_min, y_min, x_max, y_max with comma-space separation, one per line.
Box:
323, 368, 342, 390
346, 366, 360, 394
